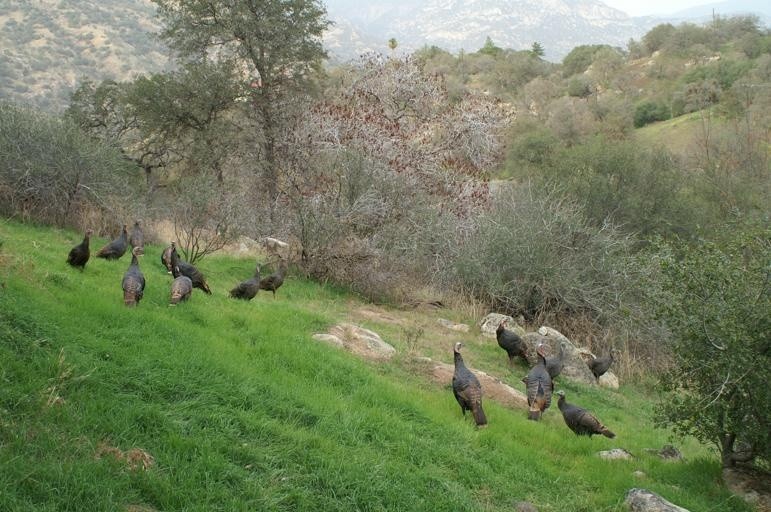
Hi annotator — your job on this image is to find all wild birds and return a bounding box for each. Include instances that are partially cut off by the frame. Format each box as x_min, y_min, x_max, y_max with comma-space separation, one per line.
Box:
452, 342, 487, 425
230, 266, 260, 301
258, 258, 288, 296
122, 246, 145, 306
96, 224, 128, 260
496, 323, 530, 367
523, 342, 616, 440
130, 219, 144, 248
66, 228, 93, 269
161, 240, 213, 308
